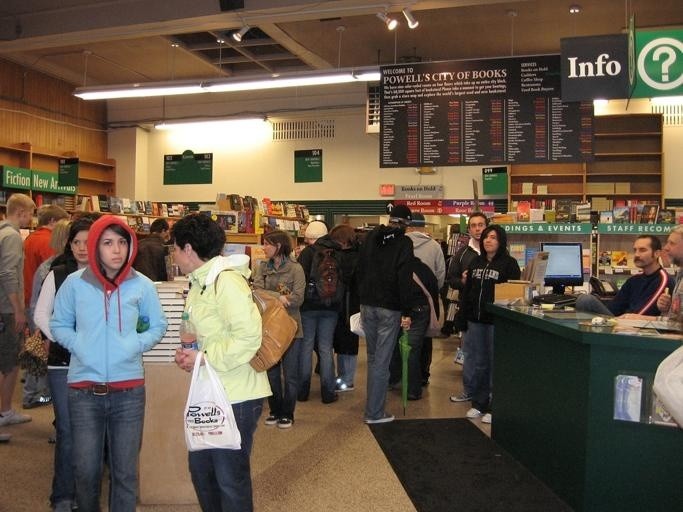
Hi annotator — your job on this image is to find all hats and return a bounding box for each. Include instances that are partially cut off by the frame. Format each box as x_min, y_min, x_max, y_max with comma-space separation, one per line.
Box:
389, 203, 413, 227
304, 221, 328, 240
408, 212, 426, 228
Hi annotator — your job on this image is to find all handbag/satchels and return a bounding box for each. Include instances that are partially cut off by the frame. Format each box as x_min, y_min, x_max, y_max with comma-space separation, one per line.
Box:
424, 299, 442, 339
213, 268, 298, 374
453, 349, 466, 366
15, 332, 49, 378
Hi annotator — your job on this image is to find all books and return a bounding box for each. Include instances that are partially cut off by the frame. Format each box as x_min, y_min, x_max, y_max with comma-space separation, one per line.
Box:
510, 197, 683, 226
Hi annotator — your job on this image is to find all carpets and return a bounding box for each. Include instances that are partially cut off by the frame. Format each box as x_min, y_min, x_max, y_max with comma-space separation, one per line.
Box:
367, 416, 576, 512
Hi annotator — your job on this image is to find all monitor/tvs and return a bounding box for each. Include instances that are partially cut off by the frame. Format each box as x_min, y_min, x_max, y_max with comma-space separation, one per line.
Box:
541, 242, 583, 295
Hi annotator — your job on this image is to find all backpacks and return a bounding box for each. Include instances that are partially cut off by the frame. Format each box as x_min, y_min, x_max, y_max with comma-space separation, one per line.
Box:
304, 243, 346, 310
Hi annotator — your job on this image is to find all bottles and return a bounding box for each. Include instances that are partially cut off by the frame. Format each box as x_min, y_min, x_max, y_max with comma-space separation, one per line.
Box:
136, 315, 150, 332
306, 280, 314, 300
179, 312, 198, 349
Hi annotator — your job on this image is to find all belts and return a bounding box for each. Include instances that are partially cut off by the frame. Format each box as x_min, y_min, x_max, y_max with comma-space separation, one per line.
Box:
81, 381, 134, 397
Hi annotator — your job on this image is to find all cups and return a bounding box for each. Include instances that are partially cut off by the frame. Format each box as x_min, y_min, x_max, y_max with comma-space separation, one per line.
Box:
545, 210, 555, 224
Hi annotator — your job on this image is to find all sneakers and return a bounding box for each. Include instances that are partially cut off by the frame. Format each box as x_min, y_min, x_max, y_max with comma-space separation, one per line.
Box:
335, 376, 343, 385
386, 377, 401, 391
278, 416, 294, 428
0, 408, 33, 428
449, 390, 473, 402
465, 407, 486, 419
407, 392, 422, 400
0, 432, 13, 441
47, 434, 57, 443
363, 412, 395, 425
481, 413, 493, 424
335, 381, 355, 393
52, 498, 74, 512
264, 415, 280, 425
22, 393, 53, 409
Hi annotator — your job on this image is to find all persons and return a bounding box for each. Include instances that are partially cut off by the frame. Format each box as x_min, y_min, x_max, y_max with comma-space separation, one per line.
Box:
575, 235, 678, 316
616, 227, 683, 331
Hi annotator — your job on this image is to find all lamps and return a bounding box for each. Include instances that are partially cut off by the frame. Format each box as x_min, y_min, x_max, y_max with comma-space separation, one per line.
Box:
230, 1, 423, 42
68, 26, 381, 101
567, 0, 581, 14
153, 93, 267, 131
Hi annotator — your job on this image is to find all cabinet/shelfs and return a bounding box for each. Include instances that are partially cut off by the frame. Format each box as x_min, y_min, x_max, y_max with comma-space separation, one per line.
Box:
587, 113, 664, 213
114, 204, 191, 240
598, 235, 682, 310
212, 197, 320, 243
31, 145, 76, 232
76, 153, 116, 217
1, 139, 33, 234
511, 159, 585, 221
